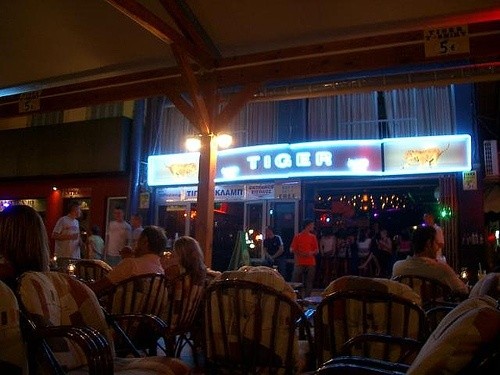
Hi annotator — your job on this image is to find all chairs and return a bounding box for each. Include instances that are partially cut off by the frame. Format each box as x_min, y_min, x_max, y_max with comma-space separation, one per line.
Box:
314, 273, 500, 367
0, 259, 222, 375
17, 272, 190, 375
312, 294, 500, 374
190, 266, 313, 375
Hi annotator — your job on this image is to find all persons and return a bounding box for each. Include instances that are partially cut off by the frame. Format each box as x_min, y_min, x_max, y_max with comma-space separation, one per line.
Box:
0, 204, 51, 295
129, 213, 144, 252
52, 201, 87, 274
89, 225, 171, 340
263, 225, 284, 272
423, 212, 445, 260
392, 226, 469, 304
88, 226, 104, 260
163, 235, 207, 358
105, 206, 132, 267
289, 220, 320, 299
321, 223, 414, 288
